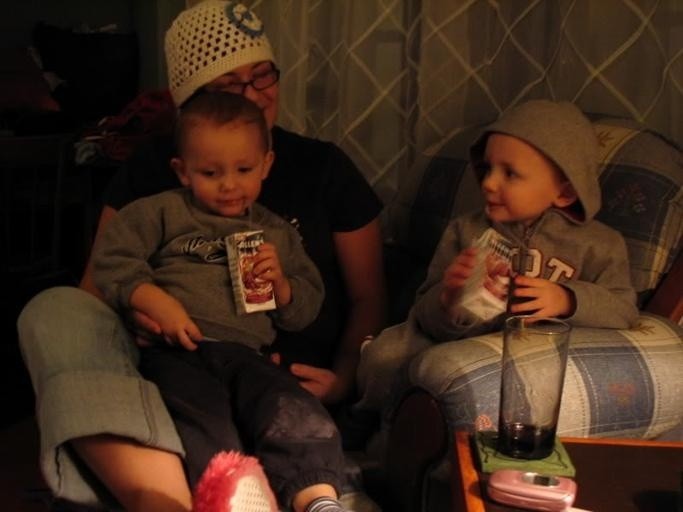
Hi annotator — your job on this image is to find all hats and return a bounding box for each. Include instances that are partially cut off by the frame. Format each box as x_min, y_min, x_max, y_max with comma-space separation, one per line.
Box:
163, 0, 277, 109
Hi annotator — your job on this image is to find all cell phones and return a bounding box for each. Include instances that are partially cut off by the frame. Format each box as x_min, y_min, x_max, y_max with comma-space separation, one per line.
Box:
487, 468, 592, 512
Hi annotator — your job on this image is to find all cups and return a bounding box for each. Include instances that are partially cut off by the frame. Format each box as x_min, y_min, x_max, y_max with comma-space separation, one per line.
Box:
500, 314, 570, 460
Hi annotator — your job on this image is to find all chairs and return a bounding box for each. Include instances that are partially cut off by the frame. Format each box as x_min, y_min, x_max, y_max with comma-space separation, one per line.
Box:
373, 110, 683, 511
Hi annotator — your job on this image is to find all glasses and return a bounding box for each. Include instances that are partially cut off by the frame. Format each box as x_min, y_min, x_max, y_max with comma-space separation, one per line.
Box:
216, 67, 279, 96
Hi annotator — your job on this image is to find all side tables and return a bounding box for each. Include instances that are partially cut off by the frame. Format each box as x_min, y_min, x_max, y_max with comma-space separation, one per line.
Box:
453, 431, 680, 511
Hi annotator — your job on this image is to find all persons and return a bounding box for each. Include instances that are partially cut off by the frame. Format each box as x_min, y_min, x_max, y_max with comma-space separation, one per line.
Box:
363, 100, 638, 420
19, 1, 386, 512
93, 87, 347, 512
